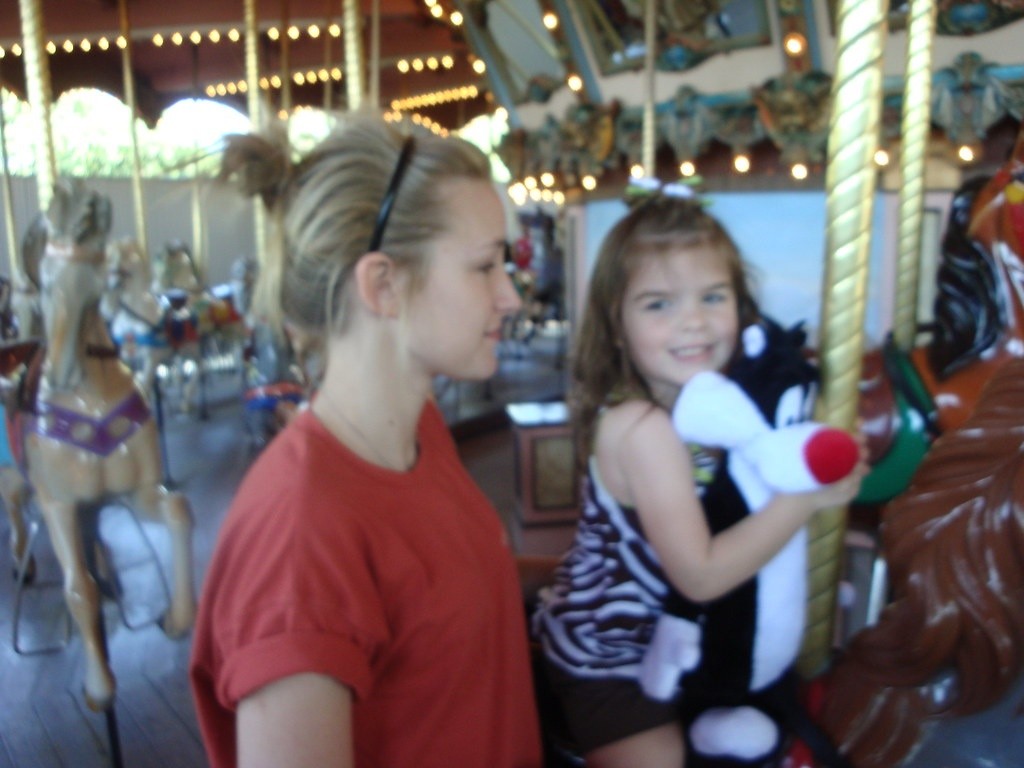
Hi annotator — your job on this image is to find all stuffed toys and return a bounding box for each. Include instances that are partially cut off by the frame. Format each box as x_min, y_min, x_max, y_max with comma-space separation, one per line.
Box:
640, 318, 862, 761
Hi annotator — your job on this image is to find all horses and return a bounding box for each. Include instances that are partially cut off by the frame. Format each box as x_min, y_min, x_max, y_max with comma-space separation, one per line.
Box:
774, 112, 1024, 768
500, 208, 566, 358
1, 176, 265, 715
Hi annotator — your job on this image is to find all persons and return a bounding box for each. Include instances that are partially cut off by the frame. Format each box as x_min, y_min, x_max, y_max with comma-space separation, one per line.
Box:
192, 114, 549, 766
523, 180, 874, 768
